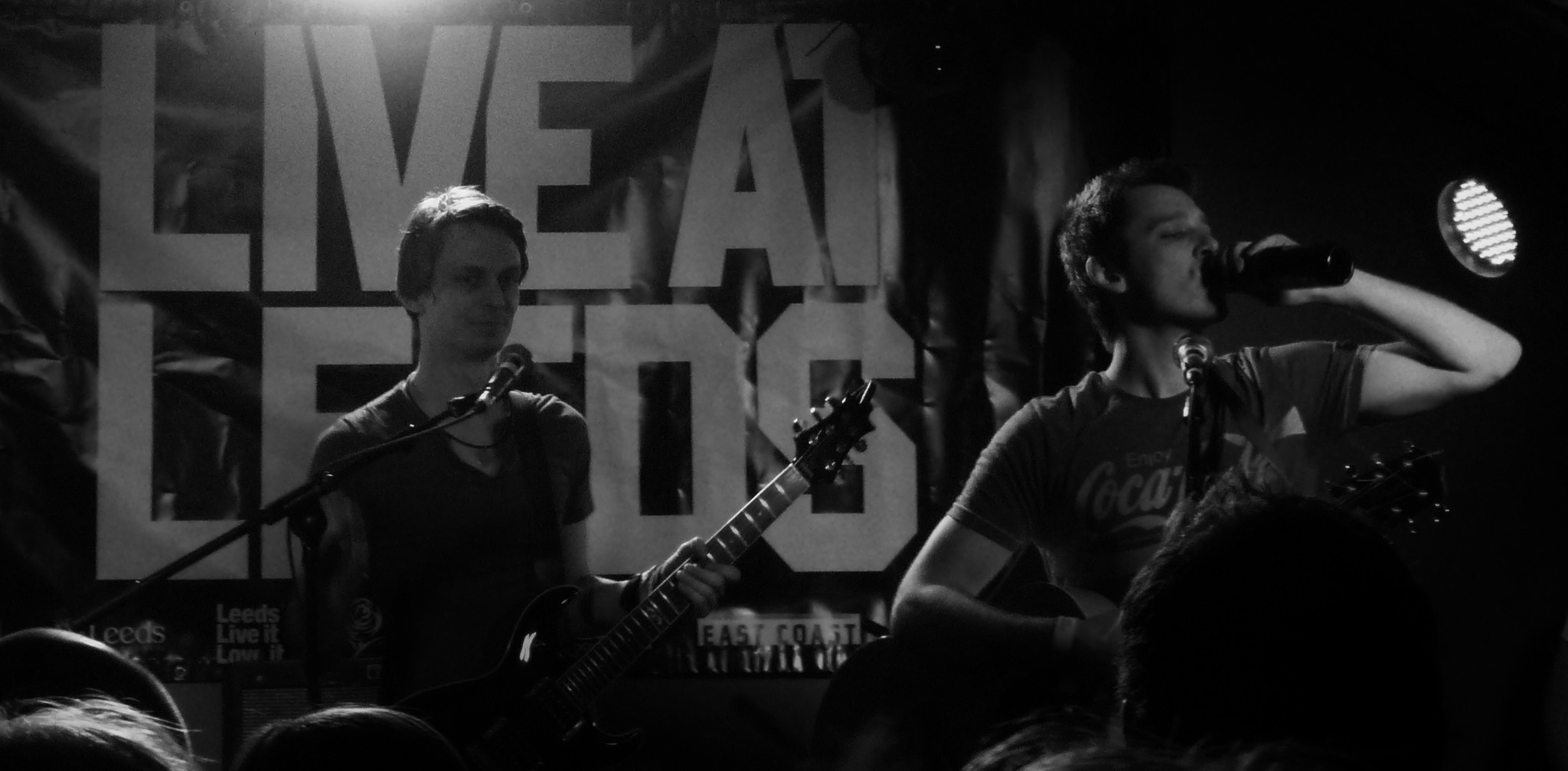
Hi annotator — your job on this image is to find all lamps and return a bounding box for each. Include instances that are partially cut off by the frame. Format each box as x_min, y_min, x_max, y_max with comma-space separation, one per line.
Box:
1438, 175, 1519, 279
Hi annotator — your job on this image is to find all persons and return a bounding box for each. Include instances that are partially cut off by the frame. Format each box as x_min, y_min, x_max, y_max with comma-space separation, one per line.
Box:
0, 186, 742, 771
890, 163, 1525, 771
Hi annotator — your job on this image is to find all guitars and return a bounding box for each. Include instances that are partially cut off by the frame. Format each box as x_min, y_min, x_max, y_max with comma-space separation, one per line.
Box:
811, 440, 1450, 771
389, 377, 877, 771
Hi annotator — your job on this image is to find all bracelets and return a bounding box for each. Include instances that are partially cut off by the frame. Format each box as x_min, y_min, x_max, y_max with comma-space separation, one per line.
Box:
620, 569, 653, 612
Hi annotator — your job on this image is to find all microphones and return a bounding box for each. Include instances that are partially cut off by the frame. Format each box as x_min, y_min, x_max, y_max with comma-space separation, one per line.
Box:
474, 343, 535, 415
1172, 337, 1214, 383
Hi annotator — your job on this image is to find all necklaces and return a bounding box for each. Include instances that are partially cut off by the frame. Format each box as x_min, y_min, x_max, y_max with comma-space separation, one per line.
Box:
405, 372, 516, 448
